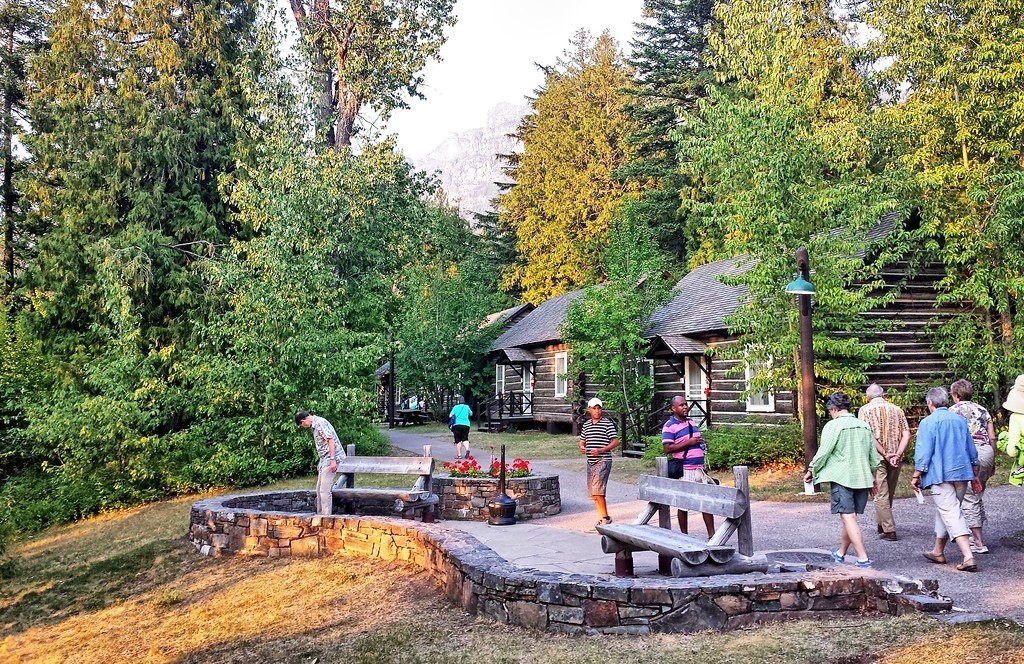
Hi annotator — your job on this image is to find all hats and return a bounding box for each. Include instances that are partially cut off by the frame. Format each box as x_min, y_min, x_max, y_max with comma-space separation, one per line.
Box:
1002, 374, 1024, 414
588, 398, 603, 407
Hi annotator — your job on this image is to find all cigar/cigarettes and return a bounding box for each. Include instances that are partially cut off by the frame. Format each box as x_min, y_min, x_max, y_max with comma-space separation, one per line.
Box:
335, 472, 336, 474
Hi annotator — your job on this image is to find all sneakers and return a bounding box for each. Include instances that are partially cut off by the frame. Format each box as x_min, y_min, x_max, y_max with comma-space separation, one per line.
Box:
969, 537, 976, 545
970, 543, 988, 553
854, 560, 872, 569
831, 548, 844, 564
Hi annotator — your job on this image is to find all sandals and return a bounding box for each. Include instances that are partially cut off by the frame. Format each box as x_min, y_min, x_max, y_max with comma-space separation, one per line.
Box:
457, 455, 462, 458
464, 450, 470, 458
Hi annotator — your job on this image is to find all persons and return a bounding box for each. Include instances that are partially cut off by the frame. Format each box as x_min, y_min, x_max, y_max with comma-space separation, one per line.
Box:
449, 396, 473, 460
858, 383, 910, 540
579, 398, 620, 526
295, 411, 346, 515
911, 388, 982, 571
1002, 374, 1024, 458
947, 379, 997, 554
804, 392, 882, 569
661, 396, 715, 539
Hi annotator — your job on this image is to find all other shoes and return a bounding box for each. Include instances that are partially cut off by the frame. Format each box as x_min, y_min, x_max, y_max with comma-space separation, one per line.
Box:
595, 516, 612, 525
956, 558, 977, 570
922, 551, 946, 564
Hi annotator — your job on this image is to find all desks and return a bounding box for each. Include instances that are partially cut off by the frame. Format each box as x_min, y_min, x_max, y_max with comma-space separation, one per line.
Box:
396, 409, 423, 426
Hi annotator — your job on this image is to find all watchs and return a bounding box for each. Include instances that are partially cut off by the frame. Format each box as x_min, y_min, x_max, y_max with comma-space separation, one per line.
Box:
330, 456, 335, 460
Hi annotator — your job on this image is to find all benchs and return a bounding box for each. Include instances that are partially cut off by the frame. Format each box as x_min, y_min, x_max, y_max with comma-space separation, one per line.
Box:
419, 415, 428, 420
595, 457, 769, 579
389, 418, 404, 422
332, 442, 439, 521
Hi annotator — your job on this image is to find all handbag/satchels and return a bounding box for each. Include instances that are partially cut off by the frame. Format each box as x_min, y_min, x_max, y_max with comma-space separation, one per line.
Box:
448, 415, 456, 429
668, 458, 683, 479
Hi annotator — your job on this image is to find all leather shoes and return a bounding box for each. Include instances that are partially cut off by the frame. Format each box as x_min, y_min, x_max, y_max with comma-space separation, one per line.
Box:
878, 532, 897, 541
877, 524, 883, 533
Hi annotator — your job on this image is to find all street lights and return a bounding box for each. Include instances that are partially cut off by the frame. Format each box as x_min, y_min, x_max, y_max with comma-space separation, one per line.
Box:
784, 245, 819, 473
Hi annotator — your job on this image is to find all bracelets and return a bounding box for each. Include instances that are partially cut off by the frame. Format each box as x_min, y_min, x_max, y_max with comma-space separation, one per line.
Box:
882, 451, 887, 457
808, 469, 812, 472
912, 476, 919, 478
